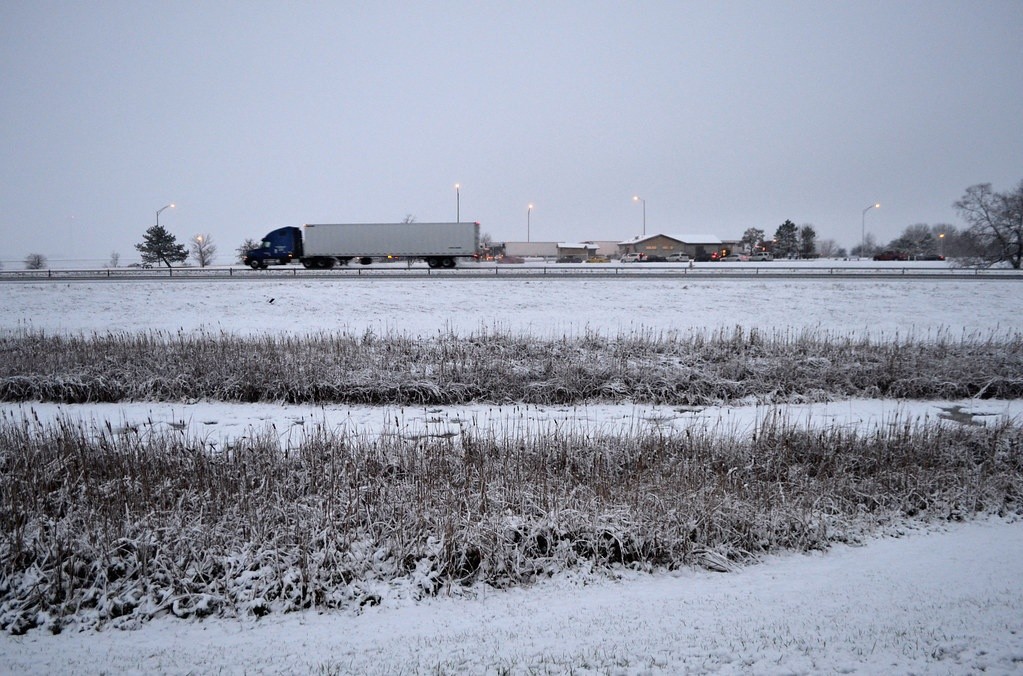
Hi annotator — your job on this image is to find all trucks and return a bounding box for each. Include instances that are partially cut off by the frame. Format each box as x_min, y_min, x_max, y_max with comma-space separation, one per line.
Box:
242, 221, 481, 269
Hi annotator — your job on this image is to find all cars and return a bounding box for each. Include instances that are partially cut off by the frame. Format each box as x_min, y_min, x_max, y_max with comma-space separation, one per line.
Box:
556, 255, 583, 264
720, 254, 743, 262
873, 250, 908, 261
910, 252, 944, 262
619, 252, 647, 262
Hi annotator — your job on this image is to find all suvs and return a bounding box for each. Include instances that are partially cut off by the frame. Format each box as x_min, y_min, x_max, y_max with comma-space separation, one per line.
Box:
749, 252, 774, 261
666, 251, 690, 262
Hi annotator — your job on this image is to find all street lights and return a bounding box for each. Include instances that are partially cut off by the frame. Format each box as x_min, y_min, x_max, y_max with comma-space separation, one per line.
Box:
938, 231, 944, 258
454, 183, 460, 223
527, 203, 532, 241
634, 196, 646, 235
154, 203, 176, 266
862, 204, 879, 258
